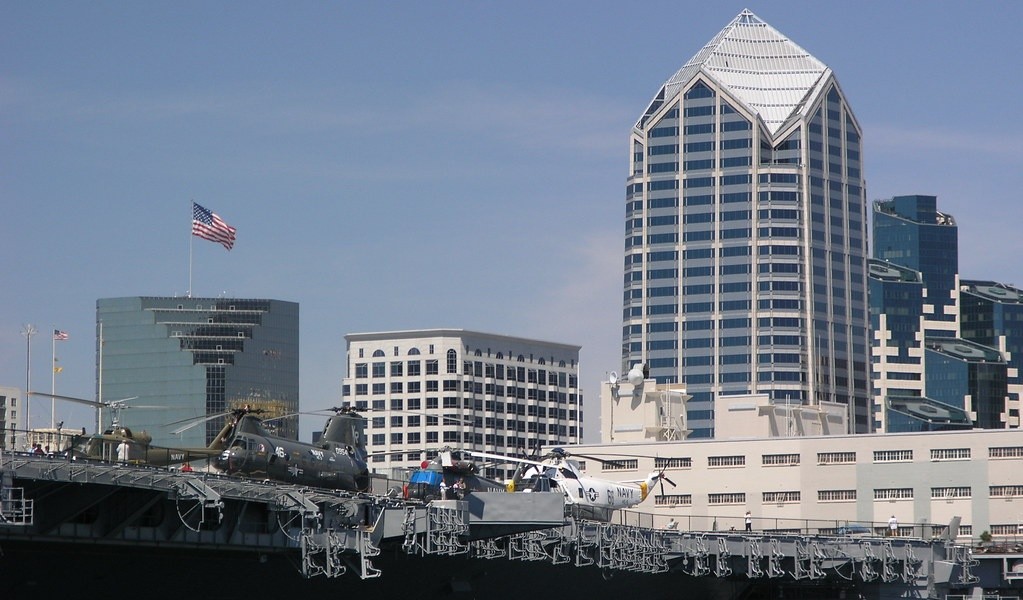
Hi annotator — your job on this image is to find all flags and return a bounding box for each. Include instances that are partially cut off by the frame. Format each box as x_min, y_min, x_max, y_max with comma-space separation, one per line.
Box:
192, 202, 236, 252
55, 330, 69, 339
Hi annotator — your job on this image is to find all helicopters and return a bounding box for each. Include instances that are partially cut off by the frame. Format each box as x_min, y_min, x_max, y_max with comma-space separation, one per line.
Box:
476, 446, 693, 523
362, 444, 563, 506
159, 403, 474, 492
22, 391, 229, 467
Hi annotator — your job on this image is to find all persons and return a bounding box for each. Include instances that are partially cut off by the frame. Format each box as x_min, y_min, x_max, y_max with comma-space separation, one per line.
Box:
440, 478, 451, 500
116, 440, 129, 466
661, 518, 675, 529
453, 477, 467, 499
28, 443, 49, 457
744, 511, 752, 531
888, 515, 897, 536
